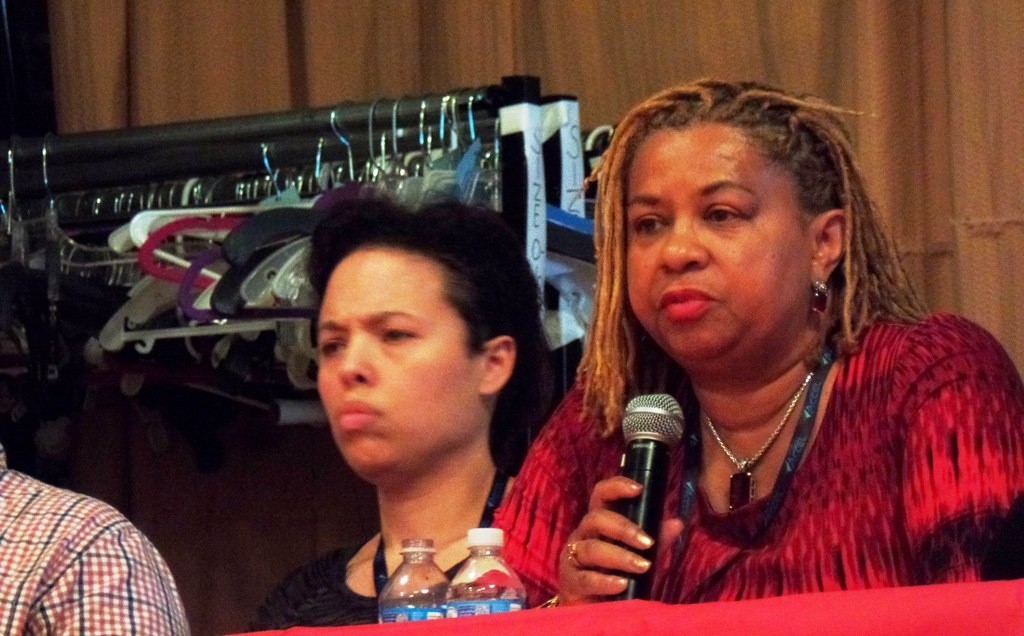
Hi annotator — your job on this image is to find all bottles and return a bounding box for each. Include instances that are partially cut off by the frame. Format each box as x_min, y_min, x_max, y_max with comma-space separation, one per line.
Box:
445, 528, 527, 619
378, 537, 453, 623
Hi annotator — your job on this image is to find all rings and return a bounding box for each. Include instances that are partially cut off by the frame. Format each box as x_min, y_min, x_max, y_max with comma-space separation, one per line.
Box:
564, 541, 584, 569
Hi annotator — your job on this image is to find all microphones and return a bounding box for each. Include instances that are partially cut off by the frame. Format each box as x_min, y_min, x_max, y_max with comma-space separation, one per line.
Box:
606, 394, 687, 604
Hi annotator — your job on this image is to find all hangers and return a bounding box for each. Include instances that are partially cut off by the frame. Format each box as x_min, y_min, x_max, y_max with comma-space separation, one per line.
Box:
0, 81, 620, 443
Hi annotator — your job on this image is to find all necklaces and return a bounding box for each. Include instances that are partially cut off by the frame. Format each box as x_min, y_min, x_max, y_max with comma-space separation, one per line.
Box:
694, 373, 816, 511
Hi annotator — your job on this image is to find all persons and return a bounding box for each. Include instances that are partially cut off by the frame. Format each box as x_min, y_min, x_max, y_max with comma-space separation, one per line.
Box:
247, 193, 550, 628
1, 428, 190, 636
487, 76, 1024, 610
